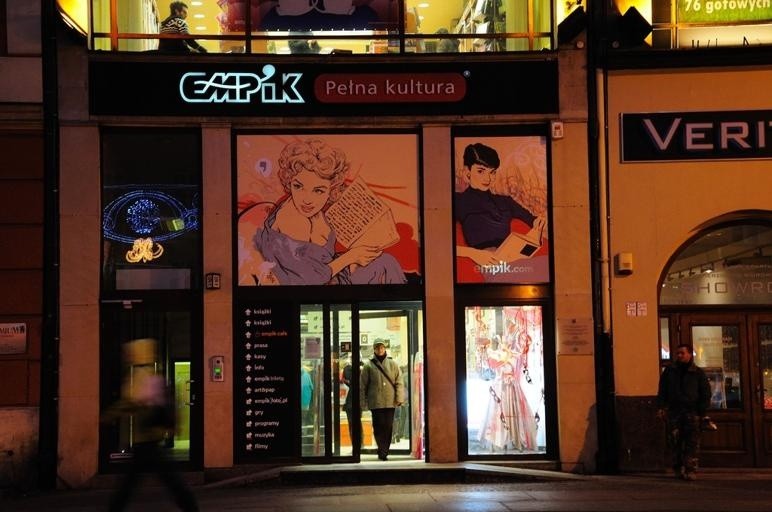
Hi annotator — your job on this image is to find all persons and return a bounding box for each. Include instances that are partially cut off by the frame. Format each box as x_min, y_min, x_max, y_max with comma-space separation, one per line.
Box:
99, 337, 202, 512
300, 365, 315, 421
457, 143, 547, 268
256, 137, 408, 285
434, 28, 462, 53
656, 344, 710, 480
342, 355, 365, 456
158, 1, 209, 54
287, 29, 322, 54
361, 338, 405, 460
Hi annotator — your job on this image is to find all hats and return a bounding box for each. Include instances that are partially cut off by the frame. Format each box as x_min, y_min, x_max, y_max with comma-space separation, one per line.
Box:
373, 338, 385, 348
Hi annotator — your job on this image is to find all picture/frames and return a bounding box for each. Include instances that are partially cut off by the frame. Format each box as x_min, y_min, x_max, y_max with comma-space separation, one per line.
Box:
449, 124, 556, 284
228, 127, 426, 295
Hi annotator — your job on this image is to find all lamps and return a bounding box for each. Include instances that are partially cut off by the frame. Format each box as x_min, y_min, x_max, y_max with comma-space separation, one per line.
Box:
665, 261, 716, 281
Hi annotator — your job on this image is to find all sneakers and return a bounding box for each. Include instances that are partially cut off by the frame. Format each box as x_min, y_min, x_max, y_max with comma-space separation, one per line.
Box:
676, 473, 683, 479
687, 473, 696, 481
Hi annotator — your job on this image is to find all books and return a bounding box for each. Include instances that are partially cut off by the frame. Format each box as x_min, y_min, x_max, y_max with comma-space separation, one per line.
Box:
321, 172, 401, 276
493, 220, 548, 265
